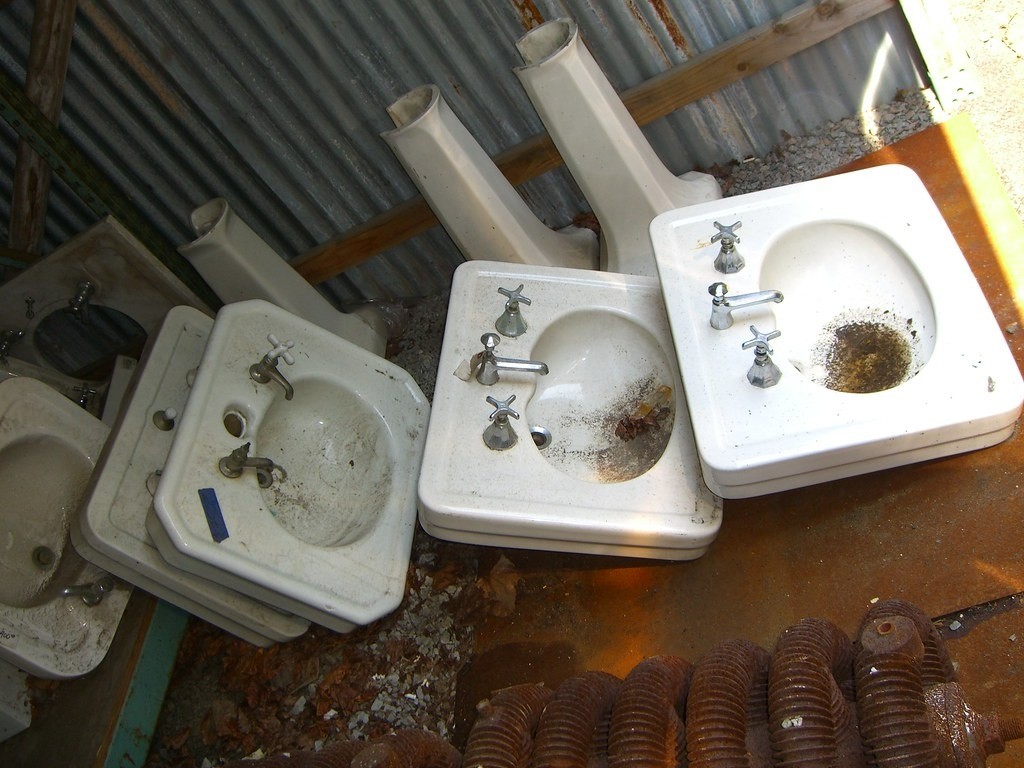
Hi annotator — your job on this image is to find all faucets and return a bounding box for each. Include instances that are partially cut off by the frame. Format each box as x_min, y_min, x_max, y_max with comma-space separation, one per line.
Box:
708, 281, 783, 331
477, 333, 549, 384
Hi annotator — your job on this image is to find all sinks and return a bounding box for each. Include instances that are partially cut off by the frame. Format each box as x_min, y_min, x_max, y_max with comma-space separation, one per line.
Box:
648, 165, 1023, 500
1, 377, 136, 682
78, 300, 427, 648
420, 259, 726, 560
